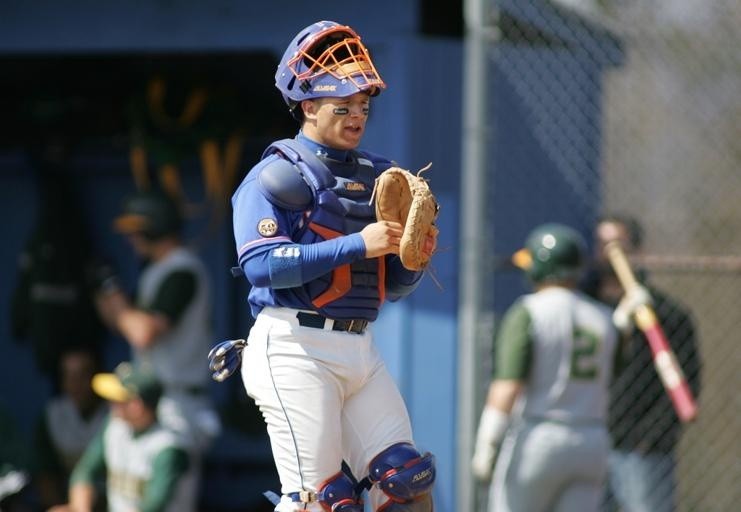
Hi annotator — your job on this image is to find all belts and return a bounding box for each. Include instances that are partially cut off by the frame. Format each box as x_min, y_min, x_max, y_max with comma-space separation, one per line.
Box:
294, 308, 372, 335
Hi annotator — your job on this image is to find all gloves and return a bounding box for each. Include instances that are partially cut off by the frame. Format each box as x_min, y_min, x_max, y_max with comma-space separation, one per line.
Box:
205, 335, 252, 385
467, 437, 500, 483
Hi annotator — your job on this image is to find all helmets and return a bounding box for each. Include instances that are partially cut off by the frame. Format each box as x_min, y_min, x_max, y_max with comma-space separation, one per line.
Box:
110, 197, 185, 239
508, 220, 593, 277
89, 357, 166, 408
274, 19, 387, 119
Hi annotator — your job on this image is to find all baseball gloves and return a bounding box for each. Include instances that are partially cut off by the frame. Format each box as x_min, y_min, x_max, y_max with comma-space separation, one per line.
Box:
374, 167, 438, 271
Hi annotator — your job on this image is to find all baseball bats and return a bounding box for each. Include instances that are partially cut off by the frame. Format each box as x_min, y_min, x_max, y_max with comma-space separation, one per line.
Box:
606, 241, 696, 422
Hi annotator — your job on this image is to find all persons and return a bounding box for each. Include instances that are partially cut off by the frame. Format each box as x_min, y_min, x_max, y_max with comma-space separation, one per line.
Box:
230, 18, 439, 512
589, 218, 704, 511
47, 360, 201, 512
30, 345, 116, 505
88, 188, 223, 475
472, 222, 618, 512
